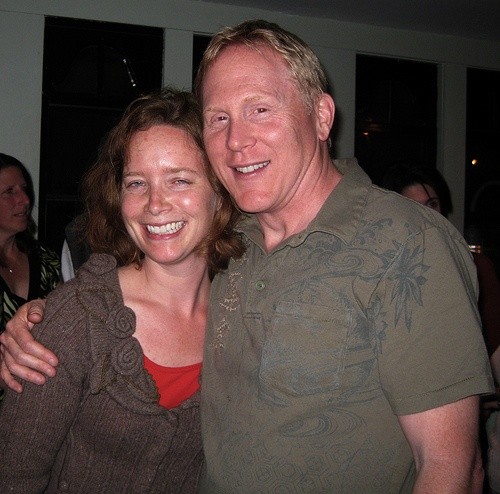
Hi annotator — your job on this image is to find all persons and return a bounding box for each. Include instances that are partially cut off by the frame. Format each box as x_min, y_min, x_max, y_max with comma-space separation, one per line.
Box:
0, 86, 243, 494
0, 151, 62, 335
0, 19, 496, 494
384, 164, 453, 219
62, 215, 93, 283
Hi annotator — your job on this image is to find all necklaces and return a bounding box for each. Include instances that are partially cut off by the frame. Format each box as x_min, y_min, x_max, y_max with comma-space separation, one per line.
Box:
0, 262, 18, 272
